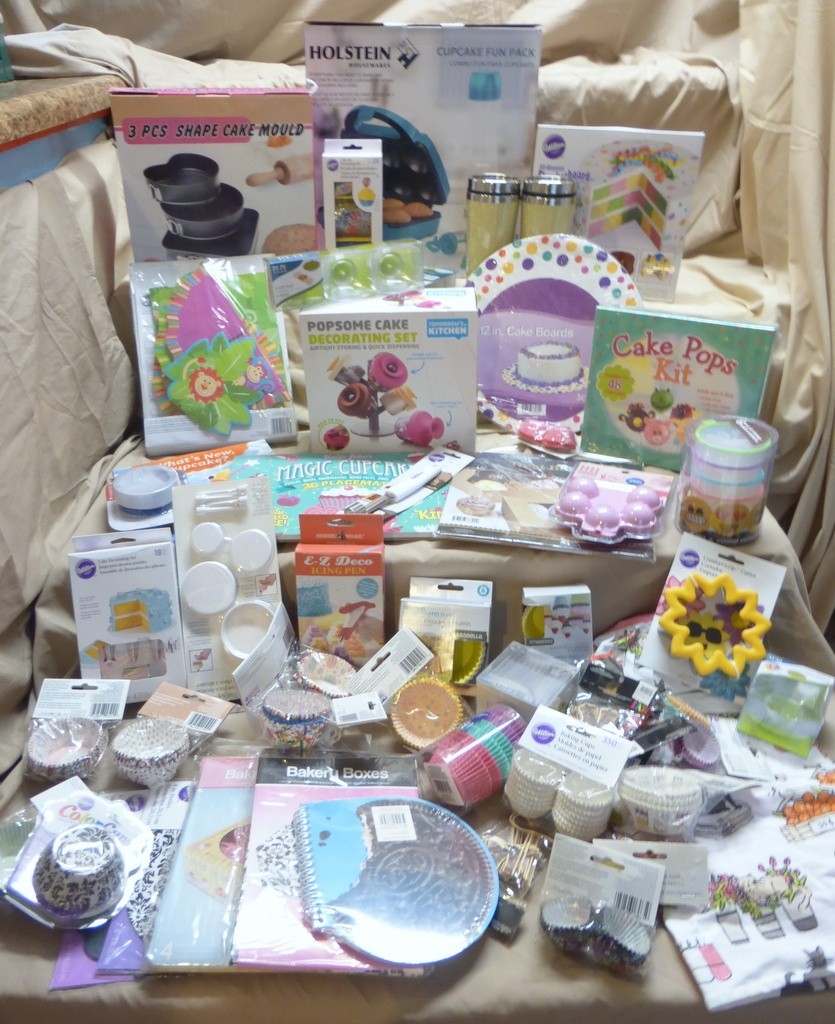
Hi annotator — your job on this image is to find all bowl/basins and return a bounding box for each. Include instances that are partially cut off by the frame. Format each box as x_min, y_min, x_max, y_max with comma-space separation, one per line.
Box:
502, 715, 721, 842
31, 823, 125, 918
25, 712, 106, 783
111, 717, 190, 786
260, 651, 357, 750
427, 704, 528, 806
390, 673, 464, 749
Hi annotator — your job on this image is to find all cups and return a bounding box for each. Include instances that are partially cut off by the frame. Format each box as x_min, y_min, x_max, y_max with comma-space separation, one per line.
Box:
520, 176, 577, 242
466, 173, 520, 278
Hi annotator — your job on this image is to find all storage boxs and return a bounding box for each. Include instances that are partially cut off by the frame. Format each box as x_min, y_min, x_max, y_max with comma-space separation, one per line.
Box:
521, 583, 593, 668
295, 512, 386, 668
320, 137, 385, 250
303, 17, 542, 243
68, 525, 185, 703
300, 284, 479, 456
110, 82, 323, 266
398, 577, 493, 697
